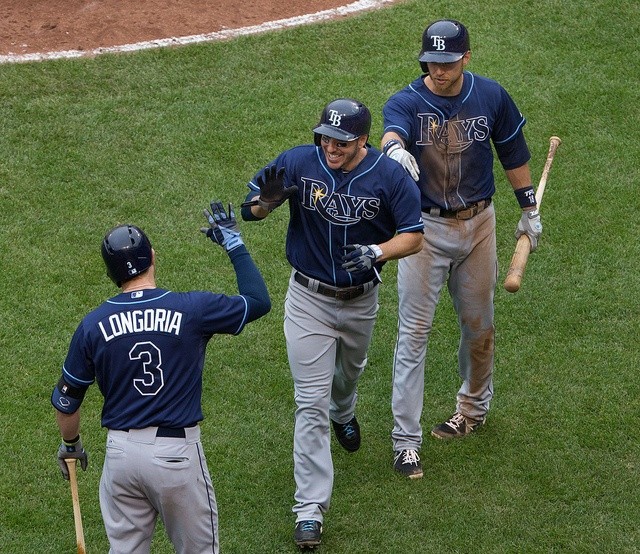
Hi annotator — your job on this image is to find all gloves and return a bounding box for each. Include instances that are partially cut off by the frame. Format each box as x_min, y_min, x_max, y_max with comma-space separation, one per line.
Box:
386, 143, 421, 182
56, 436, 89, 481
199, 199, 247, 253
257, 164, 300, 212
340, 242, 383, 274
514, 208, 543, 254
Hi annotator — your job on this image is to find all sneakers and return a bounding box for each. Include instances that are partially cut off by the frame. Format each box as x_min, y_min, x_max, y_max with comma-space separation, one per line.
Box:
331, 413, 362, 452
432, 412, 484, 441
292, 519, 324, 550
393, 448, 424, 480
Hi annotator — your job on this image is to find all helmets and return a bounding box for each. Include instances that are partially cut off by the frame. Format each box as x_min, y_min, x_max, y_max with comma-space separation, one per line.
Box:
418, 18, 471, 65
99, 224, 154, 287
312, 99, 372, 143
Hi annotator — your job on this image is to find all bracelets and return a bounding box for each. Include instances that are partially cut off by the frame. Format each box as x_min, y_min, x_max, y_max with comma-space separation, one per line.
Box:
514, 184, 537, 208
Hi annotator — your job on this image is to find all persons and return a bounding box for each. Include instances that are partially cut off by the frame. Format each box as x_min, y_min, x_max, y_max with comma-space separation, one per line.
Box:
49, 200, 271, 554
380, 20, 544, 478
240, 98, 425, 544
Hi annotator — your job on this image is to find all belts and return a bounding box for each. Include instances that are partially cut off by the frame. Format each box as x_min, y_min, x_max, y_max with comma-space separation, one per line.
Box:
117, 426, 191, 439
293, 271, 383, 300
420, 197, 492, 221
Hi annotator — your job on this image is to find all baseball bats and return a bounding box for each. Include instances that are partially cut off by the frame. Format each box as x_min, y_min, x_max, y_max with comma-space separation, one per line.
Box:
504, 136, 561, 292
63, 458, 85, 554
